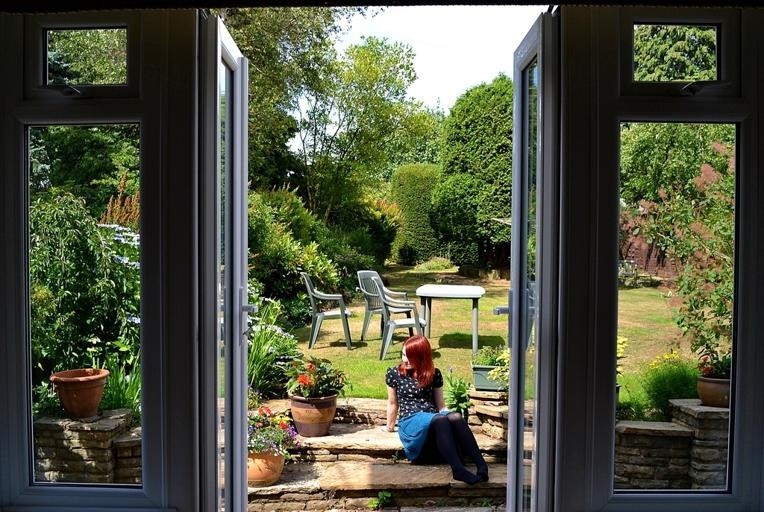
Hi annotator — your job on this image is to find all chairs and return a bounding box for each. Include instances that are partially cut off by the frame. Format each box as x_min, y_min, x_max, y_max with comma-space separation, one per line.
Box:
355, 269, 415, 341
299, 270, 353, 350
370, 276, 427, 360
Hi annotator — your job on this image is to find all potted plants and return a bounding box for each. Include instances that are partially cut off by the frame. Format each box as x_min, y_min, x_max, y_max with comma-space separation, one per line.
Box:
469, 344, 511, 392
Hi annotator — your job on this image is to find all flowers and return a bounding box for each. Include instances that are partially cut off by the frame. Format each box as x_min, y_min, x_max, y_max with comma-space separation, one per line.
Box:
282, 354, 346, 398
245, 405, 302, 461
696, 344, 732, 378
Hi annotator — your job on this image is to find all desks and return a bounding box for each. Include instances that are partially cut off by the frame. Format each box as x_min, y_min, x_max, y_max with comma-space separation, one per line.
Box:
415, 284, 485, 355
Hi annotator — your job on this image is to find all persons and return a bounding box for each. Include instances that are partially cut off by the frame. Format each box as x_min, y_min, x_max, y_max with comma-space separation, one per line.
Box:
381, 335, 489, 485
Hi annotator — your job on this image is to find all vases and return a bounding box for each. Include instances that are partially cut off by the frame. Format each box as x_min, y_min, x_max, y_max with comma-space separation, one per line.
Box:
49, 368, 110, 419
696, 375, 731, 409
246, 450, 284, 487
286, 389, 339, 437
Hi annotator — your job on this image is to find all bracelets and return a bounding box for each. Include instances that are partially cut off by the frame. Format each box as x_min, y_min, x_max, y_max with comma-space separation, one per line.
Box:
387, 427, 394, 429
